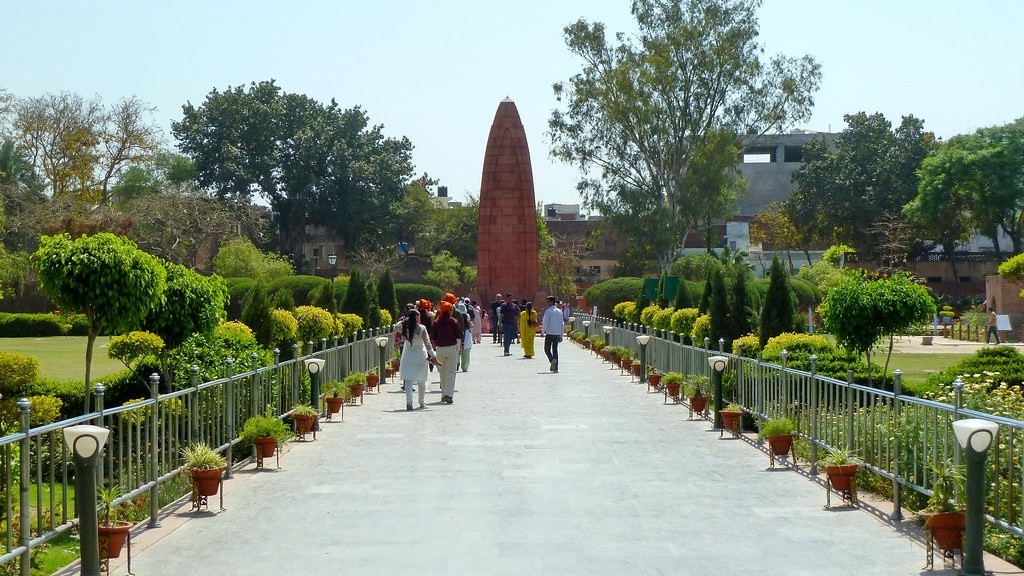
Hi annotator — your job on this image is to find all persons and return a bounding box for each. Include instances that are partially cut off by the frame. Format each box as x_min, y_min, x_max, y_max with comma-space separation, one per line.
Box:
480, 308, 489, 334
519, 302, 538, 359
487, 308, 494, 334
518, 297, 528, 311
540, 304, 547, 338
412, 294, 475, 372
495, 300, 505, 343
512, 298, 521, 343
394, 303, 415, 391
557, 298, 565, 342
555, 301, 562, 310
540, 296, 564, 372
984, 306, 1000, 344
470, 300, 481, 344
499, 292, 521, 357
489, 293, 502, 343
394, 309, 443, 410
430, 300, 464, 403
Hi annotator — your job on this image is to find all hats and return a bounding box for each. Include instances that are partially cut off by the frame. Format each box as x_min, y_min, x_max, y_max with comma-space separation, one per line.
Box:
458, 304, 467, 314
440, 300, 453, 312
420, 299, 431, 309
443, 293, 457, 304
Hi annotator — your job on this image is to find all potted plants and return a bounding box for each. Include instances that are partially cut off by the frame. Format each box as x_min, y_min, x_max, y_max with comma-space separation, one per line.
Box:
292, 357, 399, 436
718, 401, 744, 428
914, 457, 967, 551
93, 482, 132, 558
754, 411, 797, 456
686, 373, 714, 415
244, 406, 290, 460
563, 324, 662, 386
175, 442, 227, 496
815, 441, 865, 489
662, 370, 685, 397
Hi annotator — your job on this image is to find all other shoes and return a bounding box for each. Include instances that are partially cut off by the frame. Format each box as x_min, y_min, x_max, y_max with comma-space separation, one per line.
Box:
493, 341, 501, 343
457, 364, 460, 371
512, 341, 521, 344
441, 396, 453, 403
420, 405, 429, 409
523, 355, 531, 358
474, 341, 481, 344
401, 386, 416, 391
550, 359, 558, 372
504, 353, 512, 356
407, 403, 413, 410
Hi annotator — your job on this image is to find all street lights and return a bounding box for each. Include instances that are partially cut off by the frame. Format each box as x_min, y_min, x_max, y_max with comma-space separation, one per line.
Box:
582, 321, 590, 339
304, 357, 326, 410
636, 336, 650, 384
375, 336, 389, 384
951, 417, 999, 576
603, 325, 613, 345
568, 316, 576, 331
327, 252, 338, 290
63, 423, 111, 576
707, 355, 729, 431
